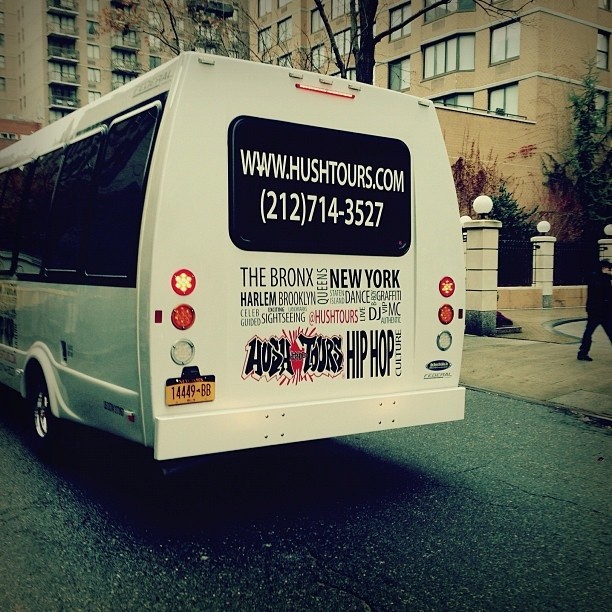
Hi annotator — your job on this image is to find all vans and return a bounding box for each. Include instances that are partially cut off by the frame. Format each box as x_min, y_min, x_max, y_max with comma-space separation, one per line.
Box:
0, 52, 466, 460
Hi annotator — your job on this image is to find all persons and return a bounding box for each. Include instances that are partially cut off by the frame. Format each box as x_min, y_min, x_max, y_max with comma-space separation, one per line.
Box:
578, 259, 612, 360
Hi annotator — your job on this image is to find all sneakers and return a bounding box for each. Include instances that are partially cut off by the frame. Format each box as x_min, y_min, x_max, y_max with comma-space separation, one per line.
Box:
577, 353, 594, 362
